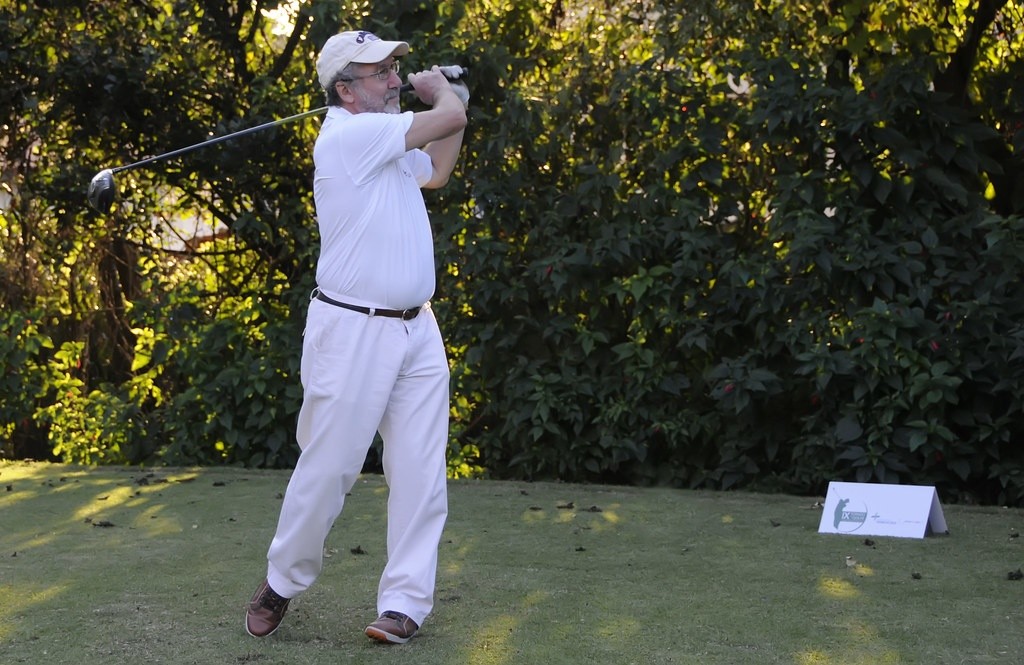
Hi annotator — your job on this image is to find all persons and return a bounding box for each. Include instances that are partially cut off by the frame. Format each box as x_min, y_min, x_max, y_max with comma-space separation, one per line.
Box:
244, 30, 470, 644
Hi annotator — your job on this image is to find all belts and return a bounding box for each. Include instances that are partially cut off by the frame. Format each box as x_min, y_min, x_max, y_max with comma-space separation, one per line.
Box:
315, 292, 421, 320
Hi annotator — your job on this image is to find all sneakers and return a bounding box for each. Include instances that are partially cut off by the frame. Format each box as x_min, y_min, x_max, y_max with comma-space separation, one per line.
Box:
365, 612, 420, 645
245, 578, 292, 639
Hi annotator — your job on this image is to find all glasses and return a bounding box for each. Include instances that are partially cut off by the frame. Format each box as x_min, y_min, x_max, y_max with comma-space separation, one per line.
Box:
333, 60, 400, 81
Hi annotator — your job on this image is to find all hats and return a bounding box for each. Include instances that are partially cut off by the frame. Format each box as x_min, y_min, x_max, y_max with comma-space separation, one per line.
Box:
316, 30, 408, 90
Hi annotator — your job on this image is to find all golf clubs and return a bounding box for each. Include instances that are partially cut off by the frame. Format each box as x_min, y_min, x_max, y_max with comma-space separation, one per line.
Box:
87, 65, 472, 209
